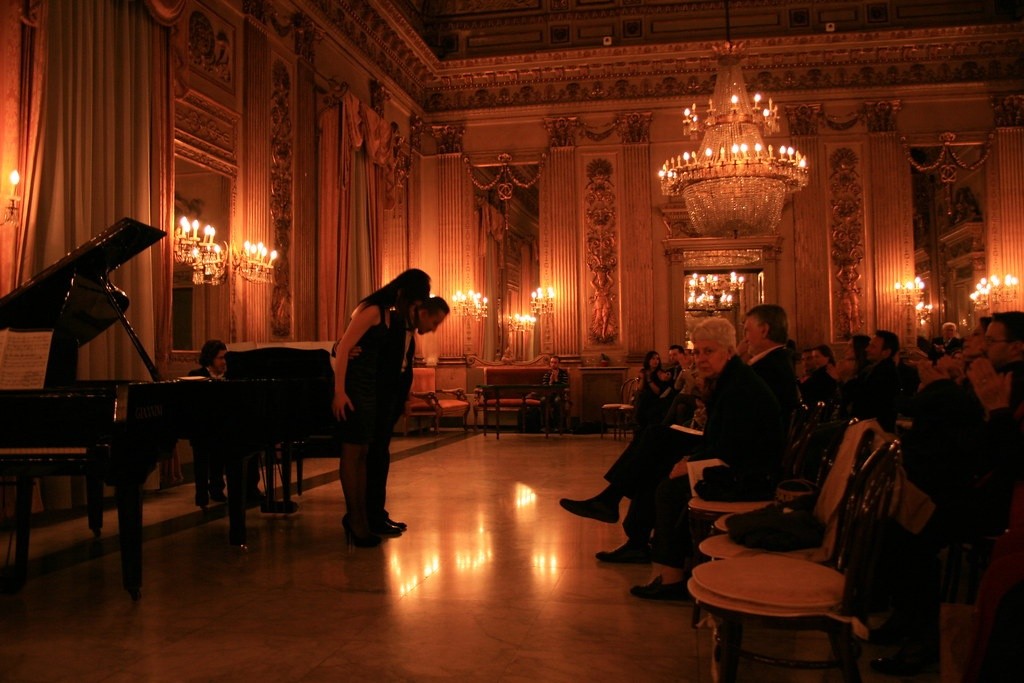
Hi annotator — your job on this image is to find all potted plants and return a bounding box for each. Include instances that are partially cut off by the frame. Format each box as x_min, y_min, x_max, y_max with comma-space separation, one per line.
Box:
600, 351, 610, 368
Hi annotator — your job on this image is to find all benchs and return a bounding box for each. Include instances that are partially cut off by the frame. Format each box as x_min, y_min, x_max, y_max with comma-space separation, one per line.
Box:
470, 363, 575, 434
399, 364, 471, 436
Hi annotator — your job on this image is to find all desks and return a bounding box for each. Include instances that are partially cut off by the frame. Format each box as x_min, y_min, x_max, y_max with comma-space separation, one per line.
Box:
476, 381, 571, 442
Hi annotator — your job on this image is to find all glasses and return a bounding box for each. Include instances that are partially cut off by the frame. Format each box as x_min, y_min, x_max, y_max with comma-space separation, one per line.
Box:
984, 336, 1016, 346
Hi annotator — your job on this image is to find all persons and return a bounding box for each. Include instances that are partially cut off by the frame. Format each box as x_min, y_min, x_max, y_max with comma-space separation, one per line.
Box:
332, 297, 449, 535
631, 344, 713, 442
591, 266, 615, 341
540, 356, 568, 433
630, 317, 781, 602
187, 340, 264, 503
836, 263, 861, 333
332, 269, 430, 550
560, 305, 796, 565
782, 311, 1024, 683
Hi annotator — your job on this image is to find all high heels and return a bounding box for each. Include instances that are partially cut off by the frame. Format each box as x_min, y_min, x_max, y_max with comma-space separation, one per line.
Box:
342, 514, 380, 546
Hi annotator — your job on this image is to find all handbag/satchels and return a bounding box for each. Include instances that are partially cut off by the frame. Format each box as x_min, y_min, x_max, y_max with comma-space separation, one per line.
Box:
727, 504, 825, 552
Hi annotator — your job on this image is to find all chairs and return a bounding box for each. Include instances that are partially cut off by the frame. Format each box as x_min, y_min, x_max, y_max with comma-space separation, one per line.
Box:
599, 376, 635, 441
613, 377, 641, 441
713, 406, 863, 536
683, 384, 840, 520
686, 431, 914, 683
696, 415, 890, 570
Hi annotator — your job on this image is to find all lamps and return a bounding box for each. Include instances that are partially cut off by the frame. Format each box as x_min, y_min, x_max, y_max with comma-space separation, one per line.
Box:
916, 300, 937, 332
234, 238, 280, 287
0, 167, 24, 229
969, 270, 1019, 317
508, 313, 538, 334
450, 286, 490, 354
685, 269, 746, 318
175, 211, 229, 288
653, 0, 814, 246
890, 275, 926, 351
529, 285, 556, 315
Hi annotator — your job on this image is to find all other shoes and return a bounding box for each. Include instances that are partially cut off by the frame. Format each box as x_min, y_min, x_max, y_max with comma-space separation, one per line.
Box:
373, 516, 407, 535
195, 490, 209, 506
631, 574, 686, 603
870, 650, 940, 677
863, 613, 896, 646
595, 540, 650, 564
208, 490, 227, 502
560, 497, 619, 523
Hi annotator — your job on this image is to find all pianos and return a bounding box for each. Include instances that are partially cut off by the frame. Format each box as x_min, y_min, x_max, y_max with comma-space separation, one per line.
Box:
0, 215, 338, 602
